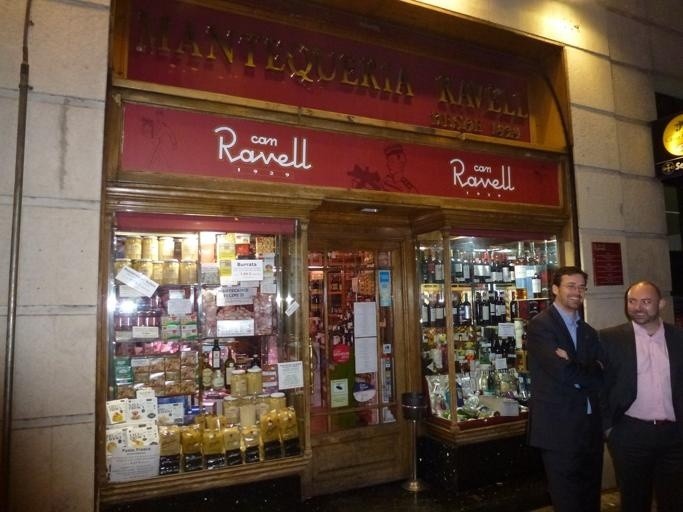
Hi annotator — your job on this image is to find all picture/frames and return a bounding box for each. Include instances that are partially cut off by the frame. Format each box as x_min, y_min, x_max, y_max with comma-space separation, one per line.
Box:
582, 234, 629, 299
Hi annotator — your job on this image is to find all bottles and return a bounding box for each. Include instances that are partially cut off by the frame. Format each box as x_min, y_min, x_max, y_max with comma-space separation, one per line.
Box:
224, 392, 288, 426
308, 247, 374, 345
417, 235, 558, 412
225, 347, 236, 391
231, 366, 261, 399
201, 362, 223, 389
252, 355, 261, 369
212, 339, 220, 371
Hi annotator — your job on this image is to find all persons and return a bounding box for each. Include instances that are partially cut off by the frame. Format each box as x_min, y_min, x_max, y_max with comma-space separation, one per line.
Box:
591, 280, 683, 511
523, 266, 610, 511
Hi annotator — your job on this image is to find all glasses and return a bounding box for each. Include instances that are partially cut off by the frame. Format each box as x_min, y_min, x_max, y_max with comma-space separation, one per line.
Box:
558, 283, 587, 292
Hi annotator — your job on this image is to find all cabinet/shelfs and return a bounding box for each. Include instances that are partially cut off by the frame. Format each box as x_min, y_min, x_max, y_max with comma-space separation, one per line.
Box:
416, 228, 564, 434
302, 246, 394, 409
102, 228, 306, 482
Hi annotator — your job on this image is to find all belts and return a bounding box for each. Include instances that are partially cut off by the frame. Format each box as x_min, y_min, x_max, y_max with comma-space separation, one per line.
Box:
646, 419, 670, 426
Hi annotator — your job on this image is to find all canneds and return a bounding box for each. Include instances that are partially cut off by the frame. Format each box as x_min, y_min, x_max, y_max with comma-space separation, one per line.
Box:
223, 367, 287, 427
114, 234, 199, 284
